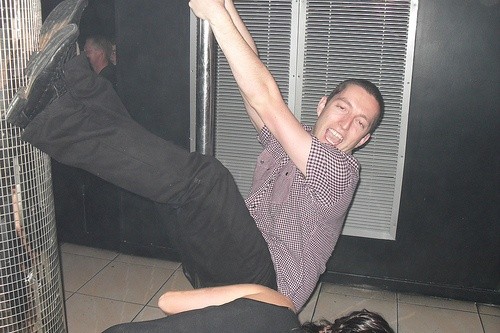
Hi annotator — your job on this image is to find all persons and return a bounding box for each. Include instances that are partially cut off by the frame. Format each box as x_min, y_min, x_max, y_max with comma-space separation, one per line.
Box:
109, 40, 117, 67
4, 0, 385, 315
83, 35, 116, 89
100, 283, 394, 333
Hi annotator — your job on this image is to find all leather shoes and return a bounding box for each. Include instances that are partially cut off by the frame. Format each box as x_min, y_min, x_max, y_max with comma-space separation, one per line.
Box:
6, 23, 80, 129
24, 0, 91, 80
